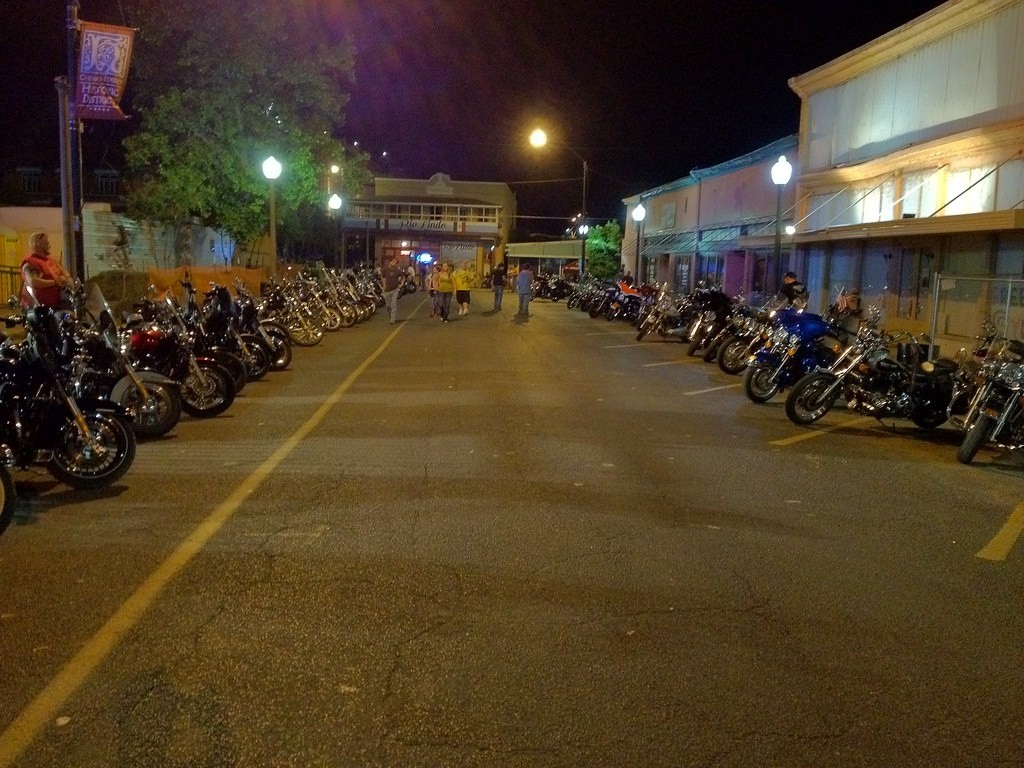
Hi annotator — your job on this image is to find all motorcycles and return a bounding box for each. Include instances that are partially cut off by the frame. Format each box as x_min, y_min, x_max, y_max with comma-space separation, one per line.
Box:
0, 254, 419, 543
507, 268, 1024, 470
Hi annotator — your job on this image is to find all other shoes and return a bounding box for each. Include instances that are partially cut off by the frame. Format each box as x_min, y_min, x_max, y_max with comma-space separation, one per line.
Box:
444, 321, 448, 324
463, 310, 468, 316
458, 311, 463, 316
440, 317, 443, 322
390, 320, 395, 325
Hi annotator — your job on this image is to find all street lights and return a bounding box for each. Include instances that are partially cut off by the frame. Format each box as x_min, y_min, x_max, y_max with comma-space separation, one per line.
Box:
262, 155, 283, 284
768, 156, 795, 300
632, 203, 647, 289
530, 125, 589, 276
327, 192, 344, 272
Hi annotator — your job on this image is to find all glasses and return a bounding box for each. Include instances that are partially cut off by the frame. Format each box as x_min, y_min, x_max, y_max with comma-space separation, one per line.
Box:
40, 233, 45, 240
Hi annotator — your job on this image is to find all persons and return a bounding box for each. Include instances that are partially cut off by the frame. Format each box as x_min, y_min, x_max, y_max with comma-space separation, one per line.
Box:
490, 262, 533, 316
615, 269, 634, 286
406, 261, 474, 323
779, 271, 810, 311
20, 232, 75, 327
380, 258, 406, 325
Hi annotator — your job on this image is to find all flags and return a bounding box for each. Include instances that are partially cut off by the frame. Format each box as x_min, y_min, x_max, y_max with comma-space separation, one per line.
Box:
836, 288, 847, 313
